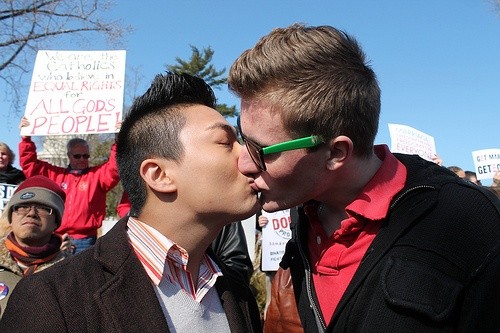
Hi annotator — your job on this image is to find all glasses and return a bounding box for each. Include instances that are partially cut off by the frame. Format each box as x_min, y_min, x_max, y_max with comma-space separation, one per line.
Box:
236, 115, 325, 172
70, 152, 91, 160
13, 205, 57, 216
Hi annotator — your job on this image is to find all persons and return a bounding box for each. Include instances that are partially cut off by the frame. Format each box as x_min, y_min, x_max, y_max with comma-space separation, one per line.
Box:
0, 70, 263, 333
227, 24, 500, 333
0, 142, 27, 186
0, 176, 65, 319
18, 116, 122, 259
116, 156, 500, 322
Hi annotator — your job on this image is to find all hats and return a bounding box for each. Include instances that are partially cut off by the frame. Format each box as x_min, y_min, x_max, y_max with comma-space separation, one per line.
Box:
7, 174, 67, 224
